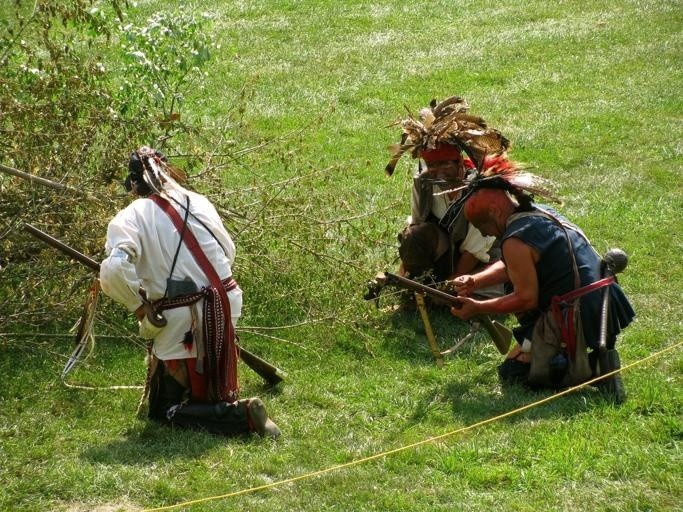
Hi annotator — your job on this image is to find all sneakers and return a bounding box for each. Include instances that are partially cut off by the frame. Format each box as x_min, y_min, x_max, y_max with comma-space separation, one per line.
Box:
589, 346, 626, 406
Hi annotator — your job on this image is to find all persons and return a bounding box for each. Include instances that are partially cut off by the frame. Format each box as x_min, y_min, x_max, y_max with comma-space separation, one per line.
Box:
98, 146, 284, 439
451, 185, 634, 405
397, 140, 513, 306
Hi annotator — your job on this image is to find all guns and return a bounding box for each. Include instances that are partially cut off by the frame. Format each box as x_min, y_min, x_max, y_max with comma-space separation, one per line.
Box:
23, 223, 289, 385
384, 272, 512, 355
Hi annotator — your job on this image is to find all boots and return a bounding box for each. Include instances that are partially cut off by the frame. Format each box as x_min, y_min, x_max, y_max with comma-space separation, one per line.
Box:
169, 394, 285, 445
